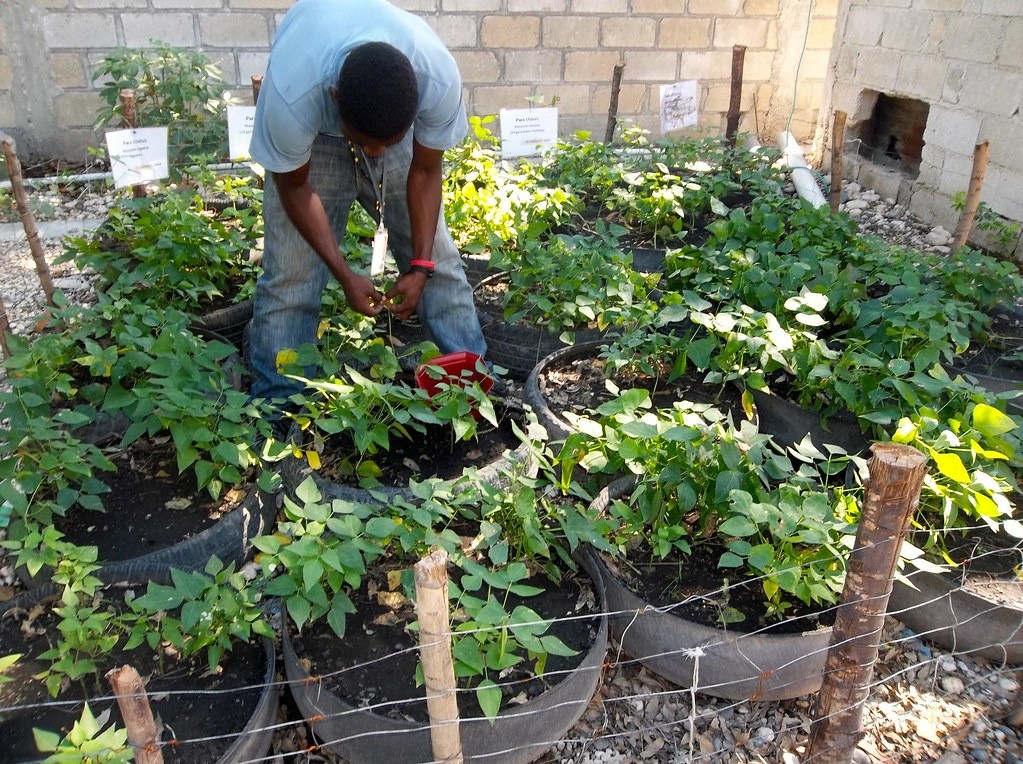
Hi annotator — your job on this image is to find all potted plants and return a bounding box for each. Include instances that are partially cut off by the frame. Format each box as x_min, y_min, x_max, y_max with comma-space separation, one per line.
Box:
0, 36, 1023, 764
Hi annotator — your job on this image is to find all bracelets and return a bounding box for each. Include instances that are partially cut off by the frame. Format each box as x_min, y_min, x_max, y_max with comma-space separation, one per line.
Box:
408, 260, 435, 279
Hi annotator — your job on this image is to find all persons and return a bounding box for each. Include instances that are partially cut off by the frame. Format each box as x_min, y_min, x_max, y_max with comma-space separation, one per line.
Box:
249, 1, 506, 449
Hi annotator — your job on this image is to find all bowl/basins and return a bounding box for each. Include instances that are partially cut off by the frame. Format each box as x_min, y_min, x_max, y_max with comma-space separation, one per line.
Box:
414, 352, 493, 421
